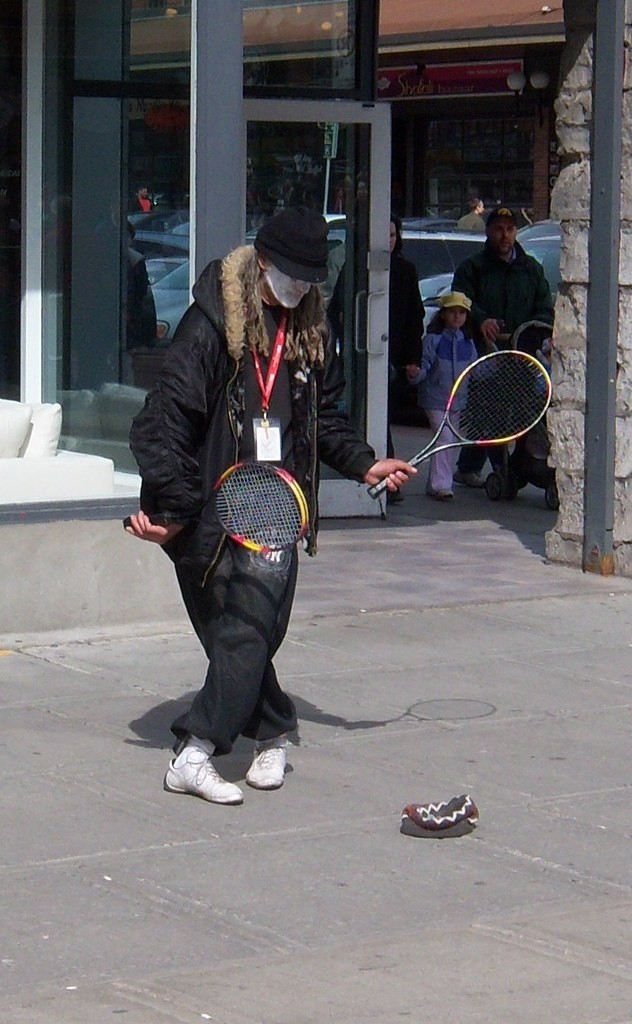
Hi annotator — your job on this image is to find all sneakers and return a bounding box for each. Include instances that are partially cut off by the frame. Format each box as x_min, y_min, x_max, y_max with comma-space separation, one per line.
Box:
245, 741, 289, 789
165, 746, 246, 805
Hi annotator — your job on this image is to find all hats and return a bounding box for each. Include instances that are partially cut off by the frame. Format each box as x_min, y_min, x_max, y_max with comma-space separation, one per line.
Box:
439, 291, 473, 313
487, 206, 520, 225
252, 206, 329, 283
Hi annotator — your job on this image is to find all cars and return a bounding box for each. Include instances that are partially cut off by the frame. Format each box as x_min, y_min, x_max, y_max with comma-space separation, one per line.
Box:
132, 210, 572, 422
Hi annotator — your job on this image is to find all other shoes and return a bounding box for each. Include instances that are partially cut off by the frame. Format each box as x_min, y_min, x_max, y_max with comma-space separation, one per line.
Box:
436, 489, 454, 499
387, 492, 405, 502
453, 468, 487, 488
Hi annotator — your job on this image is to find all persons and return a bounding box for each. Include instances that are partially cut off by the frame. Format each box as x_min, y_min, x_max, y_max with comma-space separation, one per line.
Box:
126, 206, 418, 803
123, 182, 169, 387
327, 195, 556, 500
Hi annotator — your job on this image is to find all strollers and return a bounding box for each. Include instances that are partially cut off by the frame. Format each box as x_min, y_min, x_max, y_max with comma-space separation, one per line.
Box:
473, 319, 561, 513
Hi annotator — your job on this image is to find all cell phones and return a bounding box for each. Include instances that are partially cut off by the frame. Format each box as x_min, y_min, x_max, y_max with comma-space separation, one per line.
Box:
496, 318, 504, 332
